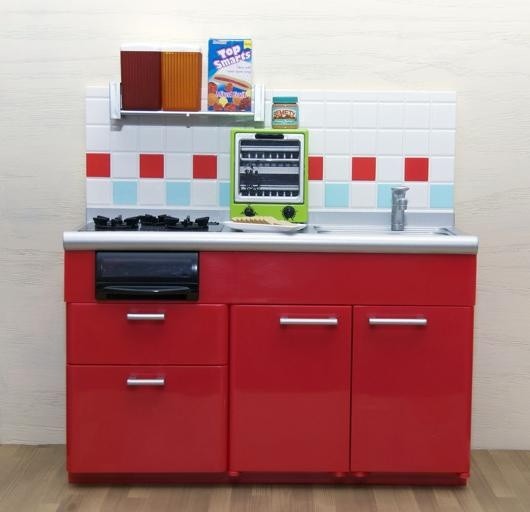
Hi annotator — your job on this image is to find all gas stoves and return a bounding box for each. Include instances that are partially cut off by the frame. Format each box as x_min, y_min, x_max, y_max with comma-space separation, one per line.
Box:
78, 212, 225, 233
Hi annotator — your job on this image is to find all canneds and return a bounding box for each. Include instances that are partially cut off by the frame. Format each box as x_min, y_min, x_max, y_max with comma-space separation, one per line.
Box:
271, 96, 299, 129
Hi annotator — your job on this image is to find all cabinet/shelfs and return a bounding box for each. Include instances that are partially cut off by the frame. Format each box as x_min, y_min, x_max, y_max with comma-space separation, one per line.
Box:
228, 249, 476, 485
64, 249, 228, 485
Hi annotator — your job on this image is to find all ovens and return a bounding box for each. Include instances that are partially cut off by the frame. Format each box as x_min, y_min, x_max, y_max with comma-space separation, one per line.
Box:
94, 251, 199, 302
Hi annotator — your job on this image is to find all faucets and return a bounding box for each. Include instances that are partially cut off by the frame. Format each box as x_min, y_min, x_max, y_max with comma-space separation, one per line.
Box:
390, 186, 410, 232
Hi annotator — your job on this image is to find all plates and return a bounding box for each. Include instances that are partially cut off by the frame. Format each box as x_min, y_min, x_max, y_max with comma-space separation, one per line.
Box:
224, 221, 307, 234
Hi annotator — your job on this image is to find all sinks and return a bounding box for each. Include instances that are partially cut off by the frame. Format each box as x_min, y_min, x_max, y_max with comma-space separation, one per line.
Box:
312, 225, 457, 236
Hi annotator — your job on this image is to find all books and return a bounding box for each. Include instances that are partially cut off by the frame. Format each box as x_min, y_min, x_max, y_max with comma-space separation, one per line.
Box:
207, 38, 255, 112
